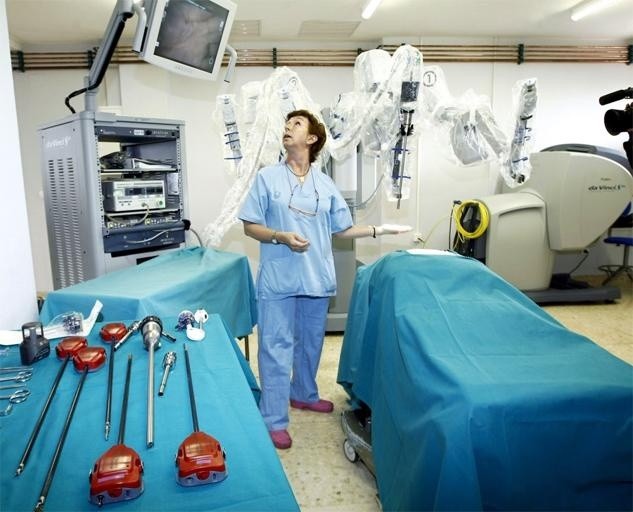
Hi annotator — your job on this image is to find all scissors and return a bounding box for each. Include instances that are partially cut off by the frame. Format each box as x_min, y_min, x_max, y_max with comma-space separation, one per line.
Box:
0, 371, 33, 403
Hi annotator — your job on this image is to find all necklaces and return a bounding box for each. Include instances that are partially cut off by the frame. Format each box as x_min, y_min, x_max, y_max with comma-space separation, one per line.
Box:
283, 159, 310, 177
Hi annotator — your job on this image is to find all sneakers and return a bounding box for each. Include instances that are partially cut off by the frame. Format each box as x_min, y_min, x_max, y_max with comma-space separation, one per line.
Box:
270, 429, 291, 449
290, 398, 333, 412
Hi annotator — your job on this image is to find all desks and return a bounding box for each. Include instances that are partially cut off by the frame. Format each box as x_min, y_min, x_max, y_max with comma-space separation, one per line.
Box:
0, 312, 301, 512
36, 245, 259, 362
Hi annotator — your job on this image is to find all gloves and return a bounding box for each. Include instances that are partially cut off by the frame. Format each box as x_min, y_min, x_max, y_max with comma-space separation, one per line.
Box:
371, 223, 412, 236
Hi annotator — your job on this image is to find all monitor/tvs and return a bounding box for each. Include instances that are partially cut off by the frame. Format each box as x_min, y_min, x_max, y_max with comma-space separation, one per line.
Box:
138, 0, 236, 82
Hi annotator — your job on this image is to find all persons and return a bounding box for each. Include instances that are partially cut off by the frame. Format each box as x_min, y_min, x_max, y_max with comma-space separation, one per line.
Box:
234, 108, 413, 448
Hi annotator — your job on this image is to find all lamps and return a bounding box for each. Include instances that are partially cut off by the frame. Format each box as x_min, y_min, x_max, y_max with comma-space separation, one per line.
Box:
360, 1, 381, 20
571, 0, 632, 21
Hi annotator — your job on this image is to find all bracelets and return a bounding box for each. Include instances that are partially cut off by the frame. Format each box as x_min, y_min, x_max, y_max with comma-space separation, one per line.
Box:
371, 225, 376, 238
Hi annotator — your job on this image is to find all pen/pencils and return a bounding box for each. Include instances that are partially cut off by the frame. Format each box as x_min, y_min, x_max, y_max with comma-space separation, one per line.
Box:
162, 332, 176, 342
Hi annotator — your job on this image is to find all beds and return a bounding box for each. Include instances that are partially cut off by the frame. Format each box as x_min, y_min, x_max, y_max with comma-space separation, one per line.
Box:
335, 246, 632, 512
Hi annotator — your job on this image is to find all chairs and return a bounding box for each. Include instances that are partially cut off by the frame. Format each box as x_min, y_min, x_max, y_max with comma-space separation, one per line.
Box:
598, 201, 633, 286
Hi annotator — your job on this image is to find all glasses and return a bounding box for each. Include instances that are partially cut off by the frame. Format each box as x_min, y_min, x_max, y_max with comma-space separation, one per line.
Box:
288, 185, 319, 216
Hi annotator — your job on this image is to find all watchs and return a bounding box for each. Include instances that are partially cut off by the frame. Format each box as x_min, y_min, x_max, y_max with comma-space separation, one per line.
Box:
270, 231, 279, 246
